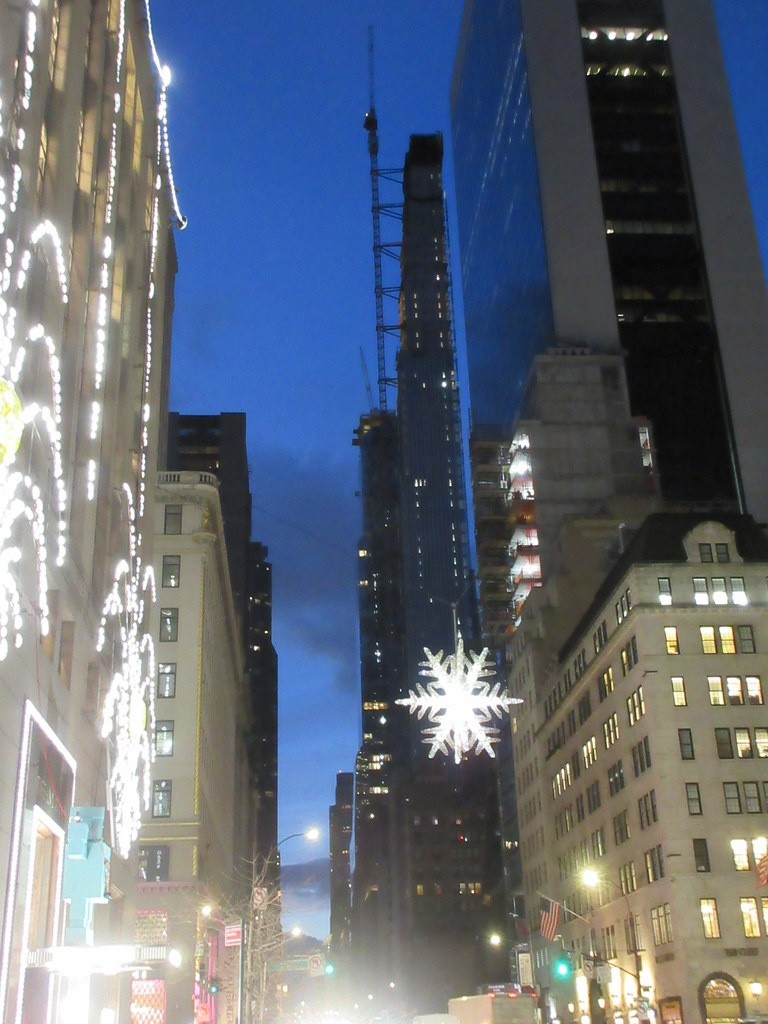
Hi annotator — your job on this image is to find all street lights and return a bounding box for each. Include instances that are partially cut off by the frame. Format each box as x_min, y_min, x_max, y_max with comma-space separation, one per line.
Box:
584, 872, 641, 998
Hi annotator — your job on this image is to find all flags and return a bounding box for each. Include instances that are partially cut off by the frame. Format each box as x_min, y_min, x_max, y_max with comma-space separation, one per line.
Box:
540, 897, 561, 941
